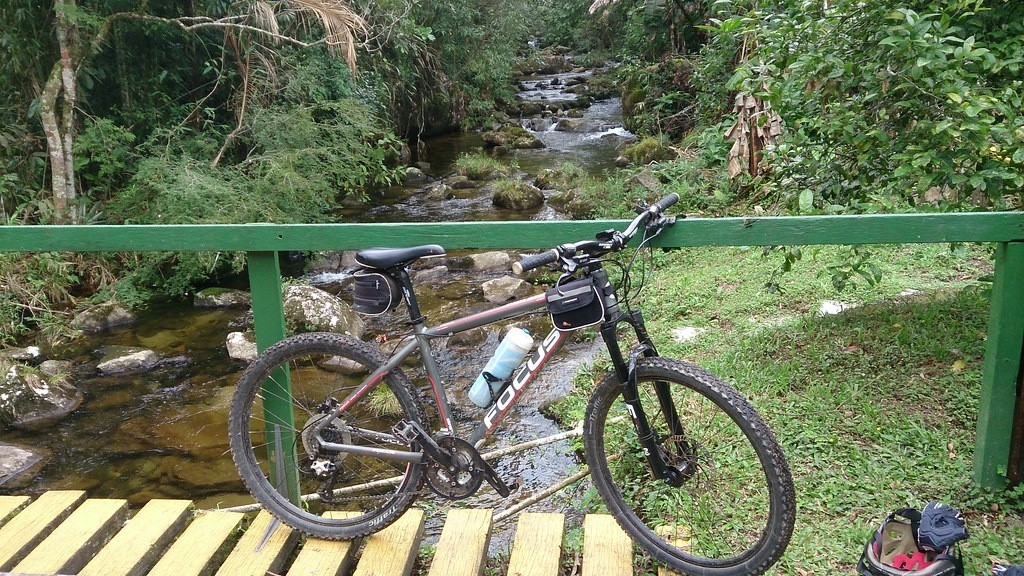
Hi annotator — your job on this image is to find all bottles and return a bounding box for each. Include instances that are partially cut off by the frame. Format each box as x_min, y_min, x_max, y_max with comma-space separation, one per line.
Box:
468, 328, 534, 409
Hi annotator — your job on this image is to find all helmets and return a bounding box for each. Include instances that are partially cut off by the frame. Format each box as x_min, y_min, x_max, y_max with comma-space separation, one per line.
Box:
860, 509, 955, 576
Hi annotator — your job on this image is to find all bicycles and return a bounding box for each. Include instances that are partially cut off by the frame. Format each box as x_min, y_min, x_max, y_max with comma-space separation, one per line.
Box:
227, 193, 798, 576
